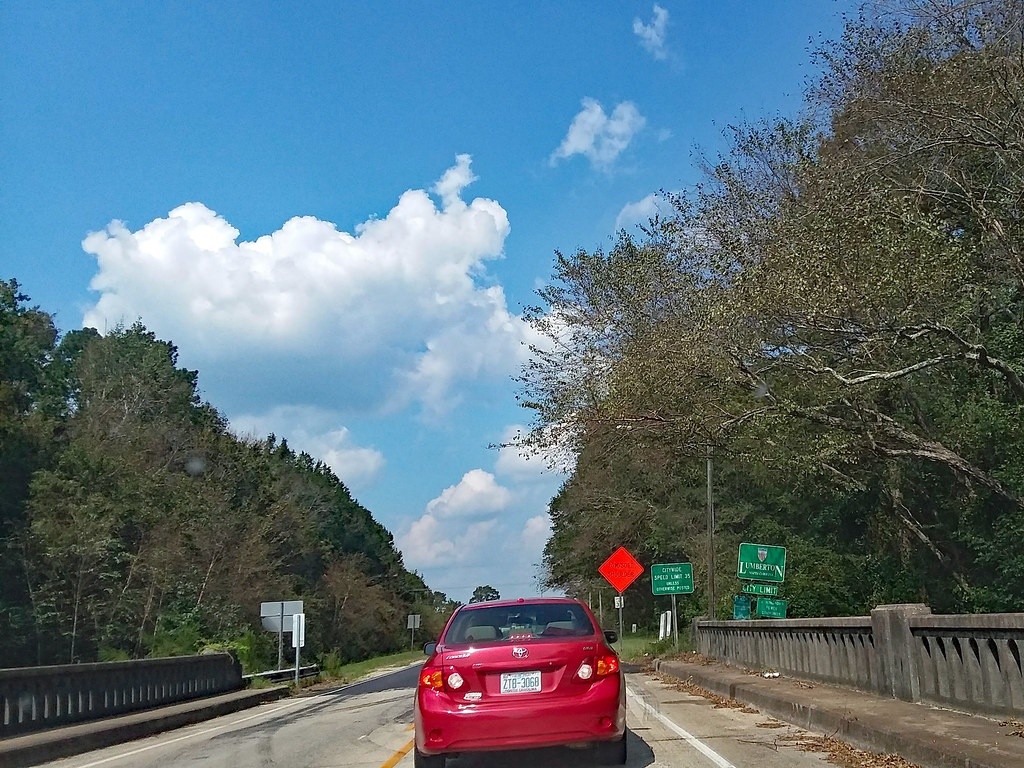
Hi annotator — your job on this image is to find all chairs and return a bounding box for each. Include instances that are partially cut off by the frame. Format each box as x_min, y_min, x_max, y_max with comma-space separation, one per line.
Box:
464, 626, 500, 641
543, 620, 580, 636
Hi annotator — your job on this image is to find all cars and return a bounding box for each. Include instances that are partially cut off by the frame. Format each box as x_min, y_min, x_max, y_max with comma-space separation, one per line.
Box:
412, 596, 627, 768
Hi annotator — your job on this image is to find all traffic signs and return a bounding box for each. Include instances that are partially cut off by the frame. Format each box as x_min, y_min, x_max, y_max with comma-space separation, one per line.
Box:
651, 563, 695, 595
